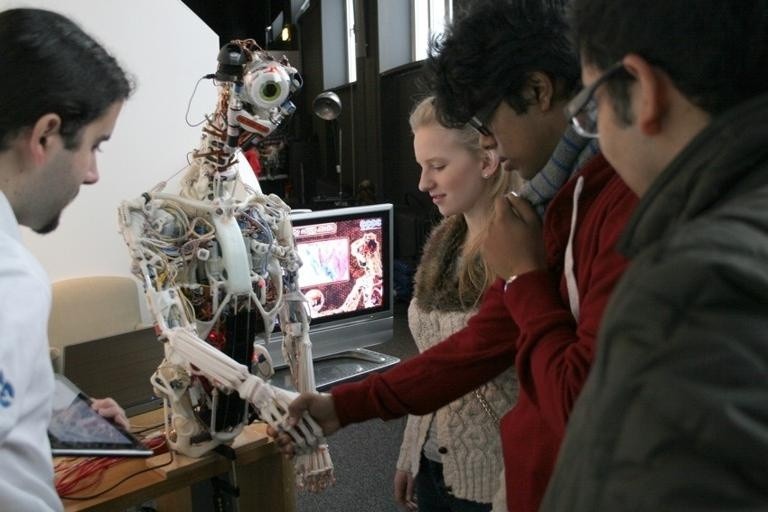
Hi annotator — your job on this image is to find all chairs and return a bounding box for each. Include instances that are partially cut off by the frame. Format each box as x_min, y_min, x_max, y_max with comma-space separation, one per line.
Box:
47, 277, 149, 371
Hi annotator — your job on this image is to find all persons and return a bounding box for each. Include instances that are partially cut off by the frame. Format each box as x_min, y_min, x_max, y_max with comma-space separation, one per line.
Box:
394, 95, 537, 512
117, 38, 337, 496
538, 2, 768, 512
268, 0, 640, 512
0, 8, 134, 510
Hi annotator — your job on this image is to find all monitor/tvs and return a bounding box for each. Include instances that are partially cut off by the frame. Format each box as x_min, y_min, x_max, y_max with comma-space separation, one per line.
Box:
248, 203, 401, 402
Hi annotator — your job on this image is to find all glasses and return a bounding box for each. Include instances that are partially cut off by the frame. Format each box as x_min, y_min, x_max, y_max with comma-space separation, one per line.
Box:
468, 61, 534, 136
561, 59, 629, 138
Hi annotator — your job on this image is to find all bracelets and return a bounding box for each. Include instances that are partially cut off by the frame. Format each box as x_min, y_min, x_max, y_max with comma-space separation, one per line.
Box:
503, 273, 517, 292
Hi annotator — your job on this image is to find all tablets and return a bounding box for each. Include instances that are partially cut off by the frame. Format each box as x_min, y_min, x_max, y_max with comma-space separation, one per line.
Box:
38, 371, 155, 458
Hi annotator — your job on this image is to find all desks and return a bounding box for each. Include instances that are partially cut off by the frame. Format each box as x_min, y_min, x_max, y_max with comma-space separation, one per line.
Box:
49, 403, 298, 512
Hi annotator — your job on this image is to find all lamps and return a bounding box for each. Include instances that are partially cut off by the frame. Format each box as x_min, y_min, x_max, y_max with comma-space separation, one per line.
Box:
312, 90, 343, 198
